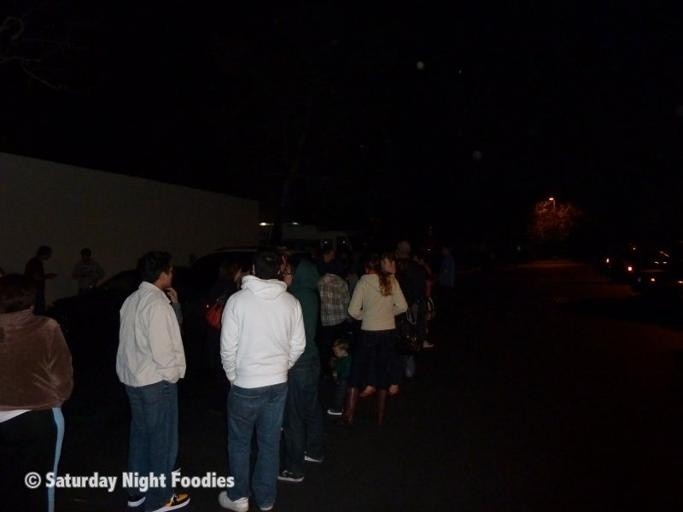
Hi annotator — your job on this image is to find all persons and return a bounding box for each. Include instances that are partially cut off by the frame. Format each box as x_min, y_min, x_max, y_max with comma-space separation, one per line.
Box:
74, 248, 103, 293
312, 224, 454, 426
264, 237, 323, 484
201, 247, 285, 330
24, 245, 58, 315
115, 252, 190, 512
216, 251, 307, 512
0, 273, 75, 512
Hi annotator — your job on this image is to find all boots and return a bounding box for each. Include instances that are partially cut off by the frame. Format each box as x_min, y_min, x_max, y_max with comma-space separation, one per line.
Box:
344, 388, 359, 424
376, 389, 388, 425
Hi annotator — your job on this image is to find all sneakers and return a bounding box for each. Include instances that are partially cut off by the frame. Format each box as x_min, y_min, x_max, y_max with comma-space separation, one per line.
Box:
155, 493, 190, 512
277, 470, 304, 483
218, 489, 249, 511
259, 502, 272, 511
387, 385, 401, 396
326, 408, 343, 416
126, 495, 145, 508
423, 340, 434, 348
359, 385, 376, 398
304, 452, 324, 463
